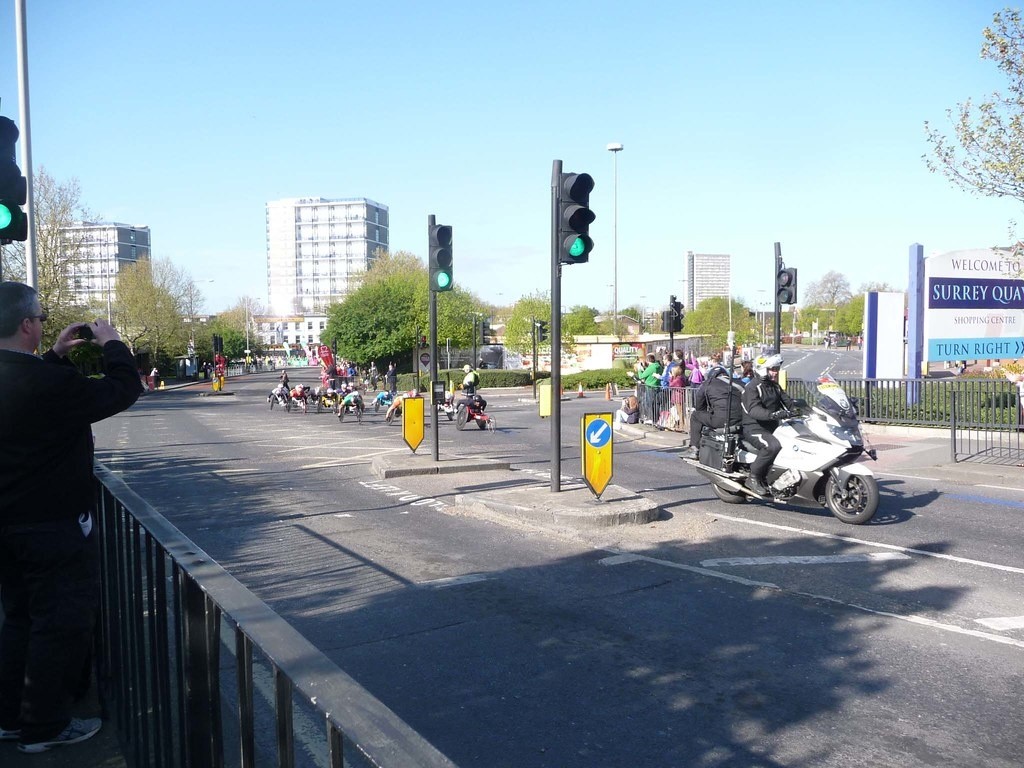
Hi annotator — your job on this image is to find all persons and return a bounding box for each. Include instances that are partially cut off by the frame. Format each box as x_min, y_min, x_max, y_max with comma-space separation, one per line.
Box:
615, 350, 754, 424
267, 358, 487, 421
740, 354, 799, 496
678, 365, 746, 462
823, 335, 863, 351
0, 279, 142, 753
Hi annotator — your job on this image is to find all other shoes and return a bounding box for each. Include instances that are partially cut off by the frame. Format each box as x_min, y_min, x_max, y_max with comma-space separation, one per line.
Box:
745, 472, 767, 496
676, 448, 699, 459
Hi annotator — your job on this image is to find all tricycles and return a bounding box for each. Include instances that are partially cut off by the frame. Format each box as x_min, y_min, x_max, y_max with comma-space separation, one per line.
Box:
456, 404, 497, 437
268, 394, 337, 414
386, 405, 402, 426
339, 403, 363, 425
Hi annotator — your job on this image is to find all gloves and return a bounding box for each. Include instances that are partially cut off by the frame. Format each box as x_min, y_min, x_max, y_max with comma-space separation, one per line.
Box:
458, 384, 464, 390
773, 410, 787, 419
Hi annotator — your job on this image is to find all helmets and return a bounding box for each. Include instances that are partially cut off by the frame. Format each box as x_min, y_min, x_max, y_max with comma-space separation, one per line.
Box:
385, 390, 391, 399
341, 384, 347, 389
444, 390, 451, 402
474, 395, 482, 402
352, 397, 358, 405
402, 393, 409, 399
315, 387, 321, 393
297, 387, 303, 394
353, 391, 360, 397
277, 384, 284, 388
327, 388, 334, 394
464, 365, 471, 373
708, 367, 729, 380
412, 389, 417, 396
752, 353, 783, 381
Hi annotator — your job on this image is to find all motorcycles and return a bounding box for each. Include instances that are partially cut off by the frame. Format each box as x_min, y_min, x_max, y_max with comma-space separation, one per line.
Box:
679, 330, 879, 523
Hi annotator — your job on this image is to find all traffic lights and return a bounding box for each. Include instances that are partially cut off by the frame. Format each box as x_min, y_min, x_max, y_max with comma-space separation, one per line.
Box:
537, 321, 549, 343
660, 312, 671, 332
429, 224, 453, 294
420, 335, 427, 348
481, 321, 490, 345
673, 302, 684, 332
778, 266, 798, 304
0, 116, 29, 242
557, 173, 596, 262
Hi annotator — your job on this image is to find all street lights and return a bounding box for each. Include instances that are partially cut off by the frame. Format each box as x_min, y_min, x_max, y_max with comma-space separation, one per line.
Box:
245, 295, 261, 357
606, 142, 624, 331
189, 277, 214, 355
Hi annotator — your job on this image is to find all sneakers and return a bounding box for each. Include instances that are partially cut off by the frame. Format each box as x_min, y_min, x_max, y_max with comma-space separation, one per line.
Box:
0, 727, 23, 740
16, 716, 102, 754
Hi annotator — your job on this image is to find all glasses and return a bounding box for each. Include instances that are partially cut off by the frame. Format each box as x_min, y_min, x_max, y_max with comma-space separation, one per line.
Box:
30, 313, 47, 321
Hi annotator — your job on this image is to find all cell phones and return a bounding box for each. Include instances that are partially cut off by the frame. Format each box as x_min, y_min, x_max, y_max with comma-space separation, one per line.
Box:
79, 327, 96, 339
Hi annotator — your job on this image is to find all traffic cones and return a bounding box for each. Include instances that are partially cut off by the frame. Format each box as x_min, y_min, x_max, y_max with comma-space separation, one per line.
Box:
576, 380, 586, 398
605, 383, 610, 400
